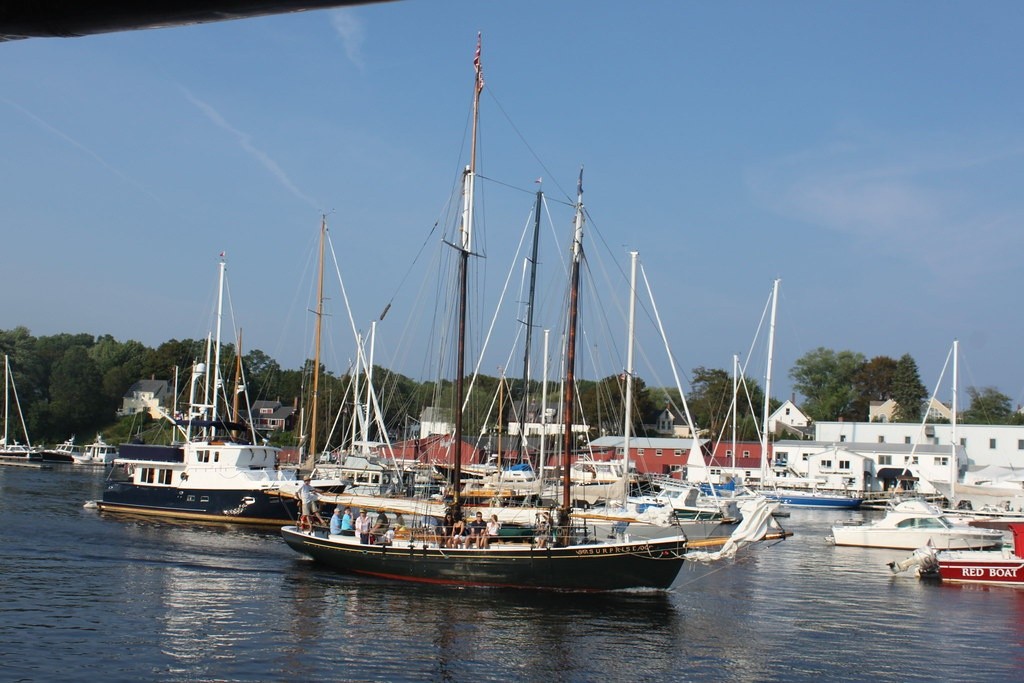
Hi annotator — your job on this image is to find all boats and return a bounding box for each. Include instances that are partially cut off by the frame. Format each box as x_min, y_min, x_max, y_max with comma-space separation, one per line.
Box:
700, 479, 863, 510
887, 539, 1024, 586
78, 435, 119, 465
43, 440, 82, 462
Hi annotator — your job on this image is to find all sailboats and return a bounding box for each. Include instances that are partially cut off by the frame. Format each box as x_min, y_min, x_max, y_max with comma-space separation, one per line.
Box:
281, 30, 794, 597
0, 354, 45, 452
87, 248, 348, 533
830, 336, 1005, 550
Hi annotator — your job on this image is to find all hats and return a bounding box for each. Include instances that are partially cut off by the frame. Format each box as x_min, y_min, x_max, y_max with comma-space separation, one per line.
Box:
334, 508, 342, 512
303, 475, 311, 481
359, 508, 366, 513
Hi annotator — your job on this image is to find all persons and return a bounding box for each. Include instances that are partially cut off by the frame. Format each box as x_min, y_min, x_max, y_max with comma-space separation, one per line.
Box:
447, 513, 467, 549
464, 512, 487, 549
294, 475, 327, 534
329, 508, 343, 535
438, 513, 454, 548
354, 508, 371, 539
340, 507, 355, 536
393, 513, 405, 530
370, 512, 389, 537
536, 512, 553, 548
480, 514, 500, 549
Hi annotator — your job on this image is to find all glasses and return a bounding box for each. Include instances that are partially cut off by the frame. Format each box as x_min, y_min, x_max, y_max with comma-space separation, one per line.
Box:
477, 516, 482, 517
447, 516, 452, 518
543, 515, 545, 517
